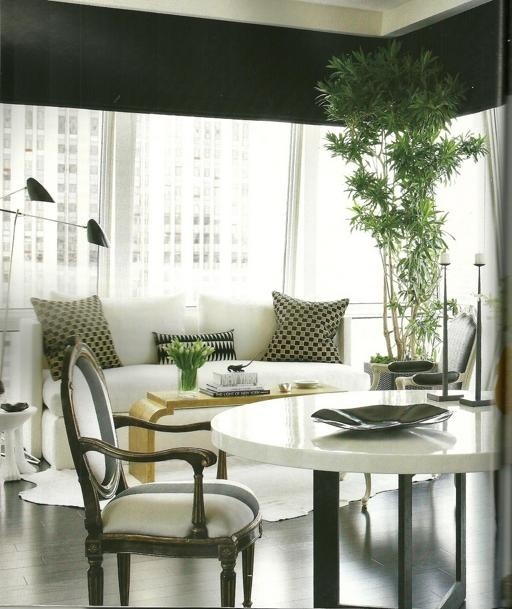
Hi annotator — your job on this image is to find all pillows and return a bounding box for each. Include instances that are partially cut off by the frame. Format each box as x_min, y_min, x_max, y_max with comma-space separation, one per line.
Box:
29, 295, 128, 383
260, 289, 350, 366
153, 327, 238, 366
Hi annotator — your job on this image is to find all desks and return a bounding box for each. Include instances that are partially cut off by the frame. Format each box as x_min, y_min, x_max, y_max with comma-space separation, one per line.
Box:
210, 387, 501, 609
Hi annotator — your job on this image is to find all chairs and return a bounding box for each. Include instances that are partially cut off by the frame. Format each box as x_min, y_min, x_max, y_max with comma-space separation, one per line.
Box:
60, 342, 265, 607
369, 311, 478, 391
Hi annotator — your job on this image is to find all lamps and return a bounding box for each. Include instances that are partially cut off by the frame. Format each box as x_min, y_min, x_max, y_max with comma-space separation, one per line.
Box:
2, 177, 57, 210
0, 202, 112, 381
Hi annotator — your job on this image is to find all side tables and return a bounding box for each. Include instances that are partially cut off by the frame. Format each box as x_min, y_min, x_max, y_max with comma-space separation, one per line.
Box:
0, 397, 39, 482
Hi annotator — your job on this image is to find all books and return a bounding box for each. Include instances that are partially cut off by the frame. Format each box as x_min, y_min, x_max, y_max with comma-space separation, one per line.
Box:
200, 383, 270, 396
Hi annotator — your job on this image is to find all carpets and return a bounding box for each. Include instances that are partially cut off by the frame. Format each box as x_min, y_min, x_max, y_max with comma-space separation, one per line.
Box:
16, 422, 443, 520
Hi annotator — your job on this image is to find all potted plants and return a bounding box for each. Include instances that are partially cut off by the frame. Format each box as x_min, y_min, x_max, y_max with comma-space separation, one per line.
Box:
163, 339, 213, 392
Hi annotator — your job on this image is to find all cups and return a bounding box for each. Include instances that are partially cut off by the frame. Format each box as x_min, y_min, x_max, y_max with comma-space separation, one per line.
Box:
279, 383, 291, 392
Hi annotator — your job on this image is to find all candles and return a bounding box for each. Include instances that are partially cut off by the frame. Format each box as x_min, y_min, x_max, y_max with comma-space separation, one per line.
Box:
473, 253, 487, 267
439, 252, 452, 265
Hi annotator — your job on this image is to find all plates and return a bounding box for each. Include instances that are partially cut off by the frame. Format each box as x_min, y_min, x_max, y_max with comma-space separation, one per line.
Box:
311, 403, 453, 433
294, 380, 319, 386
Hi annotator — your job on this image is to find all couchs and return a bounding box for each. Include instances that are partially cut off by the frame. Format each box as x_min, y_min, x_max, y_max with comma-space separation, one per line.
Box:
19, 287, 363, 469
